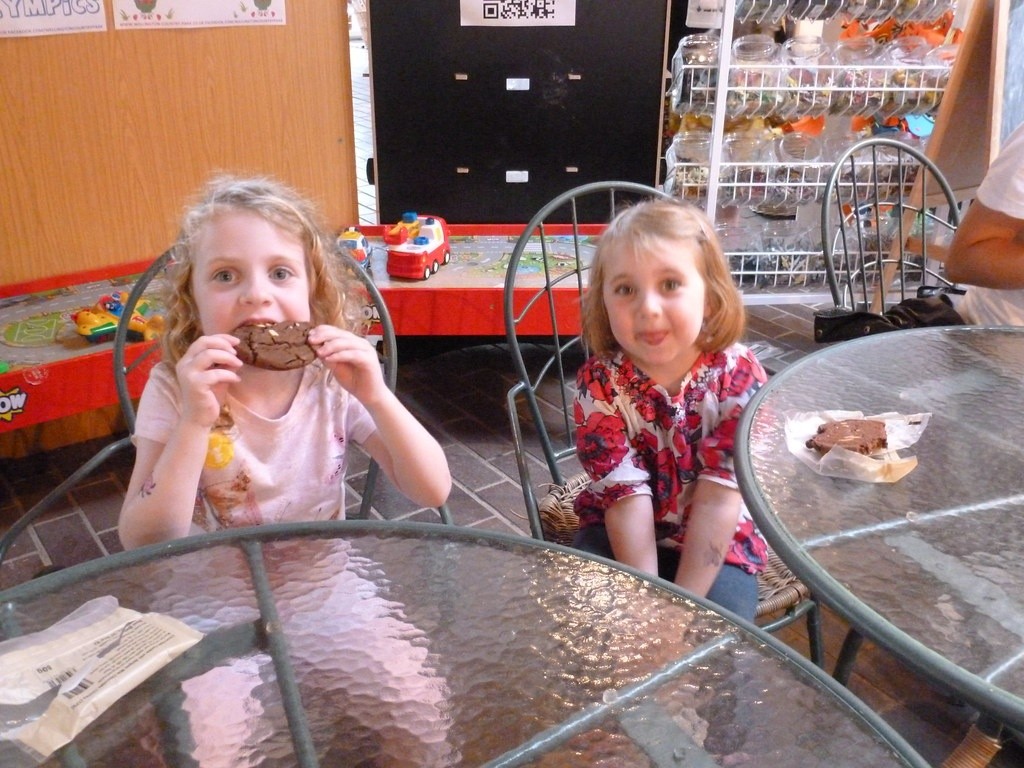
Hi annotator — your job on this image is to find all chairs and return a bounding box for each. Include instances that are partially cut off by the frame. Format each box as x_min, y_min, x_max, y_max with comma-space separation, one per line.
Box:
503, 181, 825, 680
110, 232, 459, 635
821, 138, 961, 311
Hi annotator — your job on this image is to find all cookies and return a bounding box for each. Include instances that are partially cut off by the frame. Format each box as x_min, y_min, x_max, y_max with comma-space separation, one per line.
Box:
230, 320, 318, 370
806, 419, 889, 455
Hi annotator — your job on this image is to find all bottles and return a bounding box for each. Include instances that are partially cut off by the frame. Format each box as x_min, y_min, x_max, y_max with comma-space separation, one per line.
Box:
668, 34, 968, 288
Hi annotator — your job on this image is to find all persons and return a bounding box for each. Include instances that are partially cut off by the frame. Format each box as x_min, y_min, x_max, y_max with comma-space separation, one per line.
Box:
117, 176, 453, 767
572, 201, 773, 768
945, 122, 1024, 325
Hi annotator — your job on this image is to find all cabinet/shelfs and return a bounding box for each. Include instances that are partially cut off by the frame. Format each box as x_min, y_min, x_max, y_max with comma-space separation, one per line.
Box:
650, 0, 978, 308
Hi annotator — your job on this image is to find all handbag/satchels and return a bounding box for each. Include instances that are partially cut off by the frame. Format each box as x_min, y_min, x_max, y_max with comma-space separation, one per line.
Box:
813, 285, 967, 343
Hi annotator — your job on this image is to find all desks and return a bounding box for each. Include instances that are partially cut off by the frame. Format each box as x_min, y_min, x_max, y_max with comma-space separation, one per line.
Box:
0, 518, 926, 768
733, 324, 1024, 768
